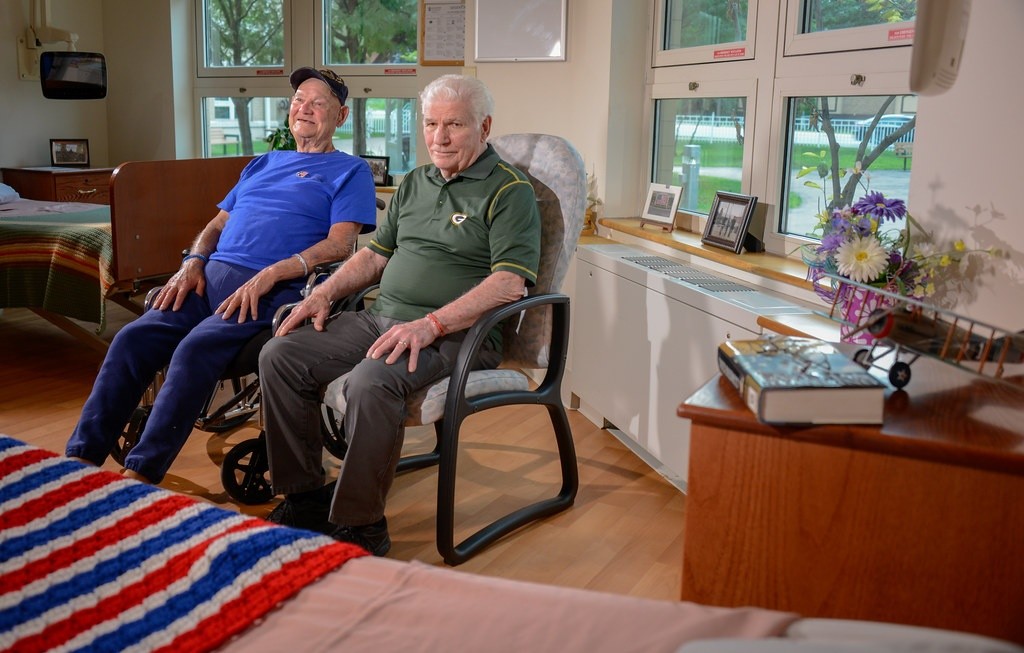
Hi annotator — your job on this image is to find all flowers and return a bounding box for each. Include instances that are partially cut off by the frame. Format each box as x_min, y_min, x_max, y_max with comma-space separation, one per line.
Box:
784, 149, 1001, 320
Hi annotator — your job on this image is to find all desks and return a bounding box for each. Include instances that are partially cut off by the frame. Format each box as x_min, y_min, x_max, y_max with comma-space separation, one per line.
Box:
677, 330, 1024, 643
757, 314, 1024, 383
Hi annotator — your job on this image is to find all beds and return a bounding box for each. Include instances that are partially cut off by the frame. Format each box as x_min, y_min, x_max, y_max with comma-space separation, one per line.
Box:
0, 429, 1024, 653
0, 155, 260, 407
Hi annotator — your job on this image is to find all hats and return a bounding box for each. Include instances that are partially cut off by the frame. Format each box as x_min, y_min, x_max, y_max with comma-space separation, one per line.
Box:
290, 66, 348, 104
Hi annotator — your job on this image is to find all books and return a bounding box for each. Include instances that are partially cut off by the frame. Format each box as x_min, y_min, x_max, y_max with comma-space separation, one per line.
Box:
718, 339, 888, 426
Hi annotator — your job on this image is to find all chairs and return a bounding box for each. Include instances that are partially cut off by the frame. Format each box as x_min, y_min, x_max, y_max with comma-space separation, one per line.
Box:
315, 132, 586, 564
110, 198, 386, 504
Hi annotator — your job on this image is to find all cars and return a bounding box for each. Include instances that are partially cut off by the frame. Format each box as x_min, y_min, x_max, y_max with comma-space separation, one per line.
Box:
852, 115, 914, 146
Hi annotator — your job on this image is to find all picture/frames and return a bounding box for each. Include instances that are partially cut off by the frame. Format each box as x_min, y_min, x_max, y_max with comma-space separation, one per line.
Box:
700, 189, 766, 254
49, 138, 90, 169
639, 183, 684, 233
359, 155, 390, 187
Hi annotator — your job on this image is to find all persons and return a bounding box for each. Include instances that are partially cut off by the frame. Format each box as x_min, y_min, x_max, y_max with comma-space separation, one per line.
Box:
719, 214, 737, 238
65, 66, 376, 486
259, 75, 542, 557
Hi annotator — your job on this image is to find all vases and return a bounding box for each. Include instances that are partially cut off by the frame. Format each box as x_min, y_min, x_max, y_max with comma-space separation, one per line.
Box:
839, 282, 896, 344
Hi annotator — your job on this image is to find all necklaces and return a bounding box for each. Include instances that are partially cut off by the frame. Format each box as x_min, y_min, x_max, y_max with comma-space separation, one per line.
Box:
399, 341, 408, 348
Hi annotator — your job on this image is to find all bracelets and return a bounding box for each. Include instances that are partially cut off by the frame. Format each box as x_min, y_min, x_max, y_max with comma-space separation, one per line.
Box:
427, 313, 446, 336
182, 253, 207, 264
293, 253, 308, 276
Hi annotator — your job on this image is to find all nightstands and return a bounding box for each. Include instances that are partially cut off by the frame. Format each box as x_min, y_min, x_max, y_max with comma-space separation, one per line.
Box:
0, 166, 116, 205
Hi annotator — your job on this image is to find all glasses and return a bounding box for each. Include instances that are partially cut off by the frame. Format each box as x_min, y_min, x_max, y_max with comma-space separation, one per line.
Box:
756, 335, 831, 372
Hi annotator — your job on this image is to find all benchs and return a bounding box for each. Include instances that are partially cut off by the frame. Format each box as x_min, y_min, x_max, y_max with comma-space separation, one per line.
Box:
894, 142, 914, 170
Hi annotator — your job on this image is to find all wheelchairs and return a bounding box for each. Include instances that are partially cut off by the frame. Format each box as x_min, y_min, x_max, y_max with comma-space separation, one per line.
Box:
104, 255, 369, 507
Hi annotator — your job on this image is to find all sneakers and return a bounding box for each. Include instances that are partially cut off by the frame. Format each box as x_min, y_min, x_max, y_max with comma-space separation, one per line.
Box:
264, 491, 333, 534
331, 516, 391, 558
